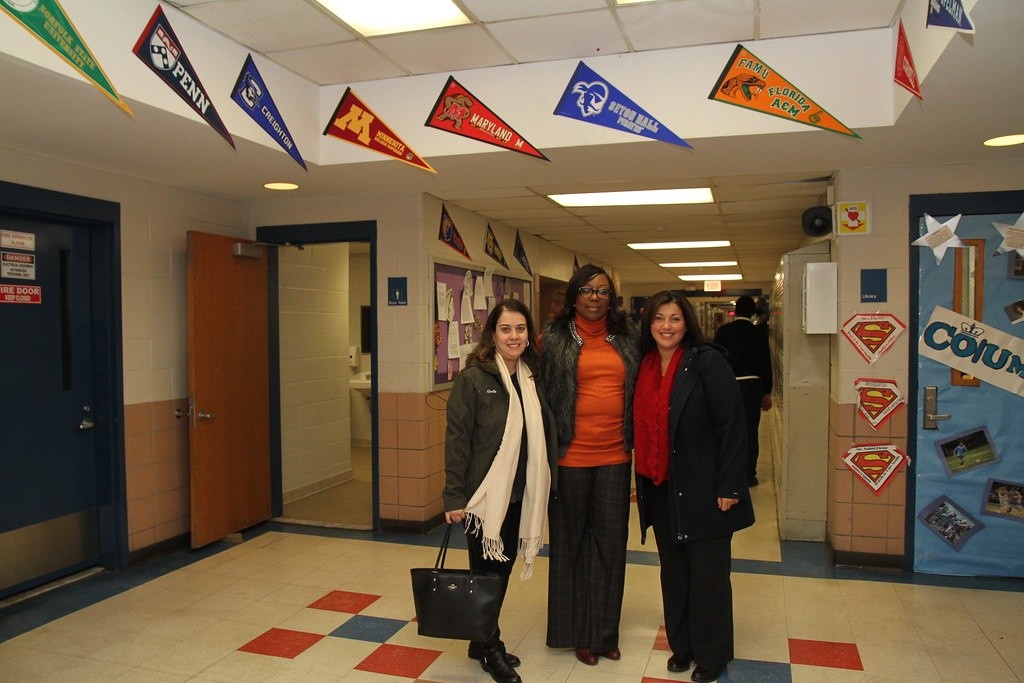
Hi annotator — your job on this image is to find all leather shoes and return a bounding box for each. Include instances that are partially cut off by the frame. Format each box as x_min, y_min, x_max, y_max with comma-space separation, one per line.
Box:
574, 647, 598, 665
599, 645, 620, 659
468, 639, 521, 667
691, 662, 727, 683
481, 644, 522, 683
667, 651, 694, 672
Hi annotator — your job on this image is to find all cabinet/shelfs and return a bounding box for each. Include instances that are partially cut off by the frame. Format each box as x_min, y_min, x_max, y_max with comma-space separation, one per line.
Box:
766, 238, 828, 542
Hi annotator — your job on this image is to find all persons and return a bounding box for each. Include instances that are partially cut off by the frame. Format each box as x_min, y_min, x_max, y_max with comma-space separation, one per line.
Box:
533, 264, 642, 666
443, 299, 558, 683
713, 295, 773, 488
633, 290, 755, 683
993, 486, 1023, 514
953, 440, 968, 467
939, 519, 969, 541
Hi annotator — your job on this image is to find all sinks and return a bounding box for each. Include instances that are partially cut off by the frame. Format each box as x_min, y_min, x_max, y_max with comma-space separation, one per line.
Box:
348, 370, 371, 398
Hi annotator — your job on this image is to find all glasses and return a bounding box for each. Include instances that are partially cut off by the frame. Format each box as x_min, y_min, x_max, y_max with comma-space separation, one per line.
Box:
579, 287, 611, 300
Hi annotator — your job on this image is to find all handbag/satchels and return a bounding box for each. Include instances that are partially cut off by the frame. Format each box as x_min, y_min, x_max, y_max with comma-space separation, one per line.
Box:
410, 516, 501, 642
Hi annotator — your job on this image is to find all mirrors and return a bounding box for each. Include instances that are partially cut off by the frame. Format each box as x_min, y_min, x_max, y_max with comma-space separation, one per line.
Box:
359, 305, 370, 354
